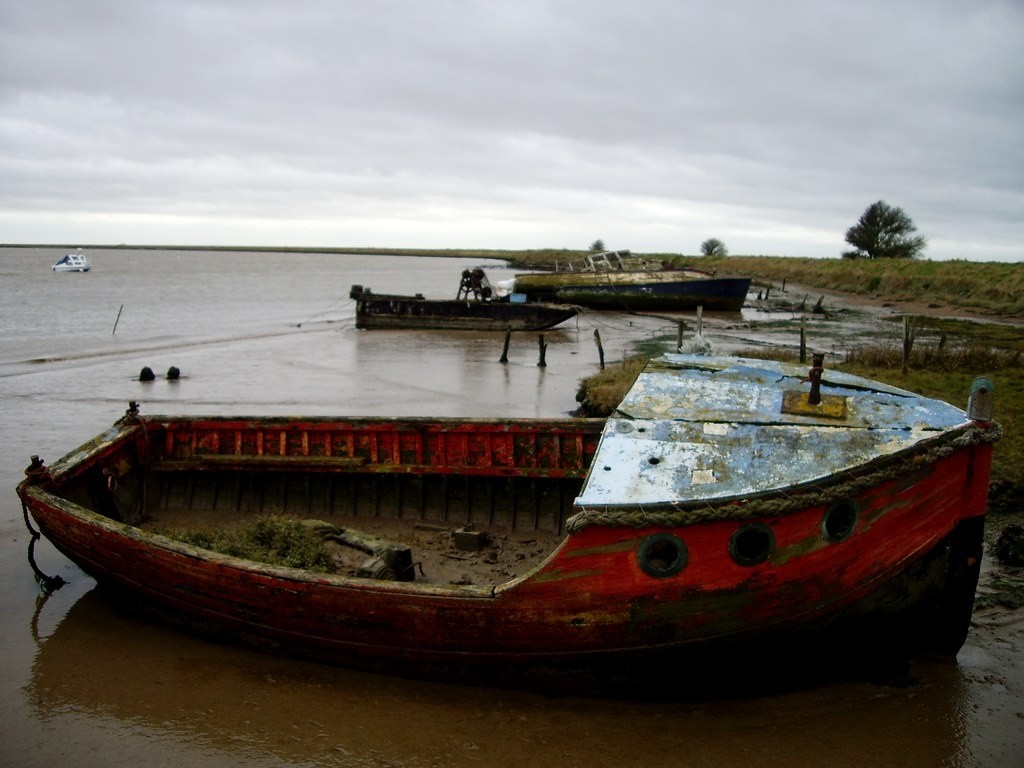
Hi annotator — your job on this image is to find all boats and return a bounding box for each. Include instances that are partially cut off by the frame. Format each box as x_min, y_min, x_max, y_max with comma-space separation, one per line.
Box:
14, 328, 1004, 680
350, 268, 586, 341
51, 246, 93, 273
511, 249, 754, 317
